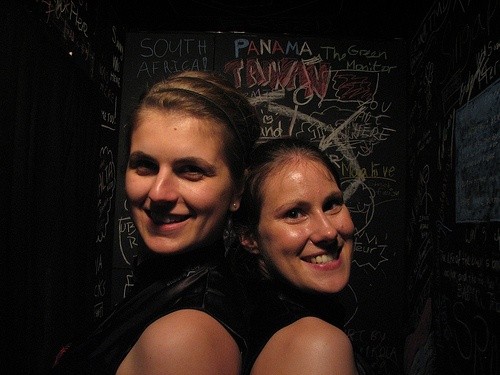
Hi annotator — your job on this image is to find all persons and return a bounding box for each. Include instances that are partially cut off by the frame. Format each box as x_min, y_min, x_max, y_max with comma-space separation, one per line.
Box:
228, 136, 395, 375
54, 69, 259, 375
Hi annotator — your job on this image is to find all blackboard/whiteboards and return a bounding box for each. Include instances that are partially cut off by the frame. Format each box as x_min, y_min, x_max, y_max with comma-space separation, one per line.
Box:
0, 0, 500, 375
